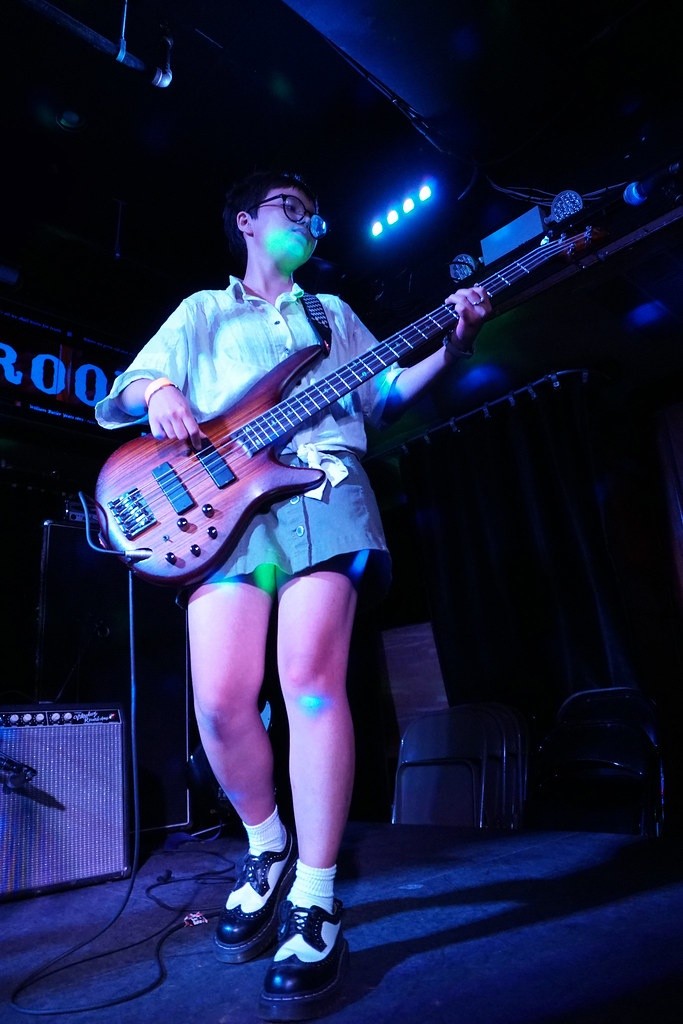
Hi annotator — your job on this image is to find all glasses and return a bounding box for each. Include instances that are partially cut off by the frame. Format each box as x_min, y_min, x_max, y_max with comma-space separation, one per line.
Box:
247, 194, 330, 240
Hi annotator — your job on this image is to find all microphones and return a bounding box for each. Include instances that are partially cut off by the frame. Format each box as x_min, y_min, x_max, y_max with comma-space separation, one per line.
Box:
7, 771, 35, 789
623, 161, 683, 206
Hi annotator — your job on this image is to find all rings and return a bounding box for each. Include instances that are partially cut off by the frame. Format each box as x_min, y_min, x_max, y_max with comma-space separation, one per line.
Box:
472, 297, 485, 307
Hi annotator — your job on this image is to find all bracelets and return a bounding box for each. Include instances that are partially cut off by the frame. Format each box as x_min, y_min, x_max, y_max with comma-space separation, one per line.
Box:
443, 330, 476, 361
144, 377, 183, 408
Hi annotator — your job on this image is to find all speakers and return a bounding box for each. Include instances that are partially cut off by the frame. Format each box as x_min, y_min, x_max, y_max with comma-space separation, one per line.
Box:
30, 520, 193, 832
0, 701, 131, 904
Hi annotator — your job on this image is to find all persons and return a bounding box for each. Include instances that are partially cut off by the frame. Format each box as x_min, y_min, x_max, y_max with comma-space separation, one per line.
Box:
94, 169, 492, 1018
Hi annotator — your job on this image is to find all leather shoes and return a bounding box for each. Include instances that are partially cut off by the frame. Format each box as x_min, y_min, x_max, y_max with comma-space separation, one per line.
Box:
257, 896, 350, 1021
211, 822, 299, 962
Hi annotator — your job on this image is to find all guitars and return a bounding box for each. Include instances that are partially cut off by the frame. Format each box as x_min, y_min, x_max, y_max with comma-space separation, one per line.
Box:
96, 222, 609, 588
